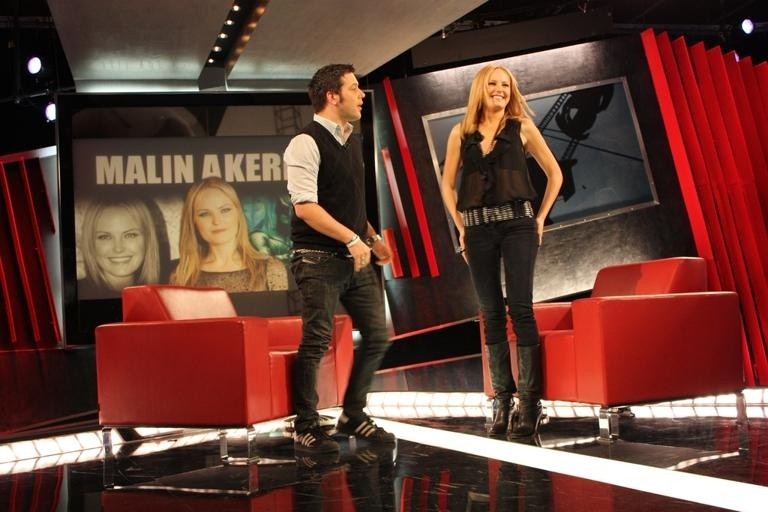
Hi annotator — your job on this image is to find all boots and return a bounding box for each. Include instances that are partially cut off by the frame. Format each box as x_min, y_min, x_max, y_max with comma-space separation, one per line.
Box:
484, 343, 515, 437
508, 343, 543, 440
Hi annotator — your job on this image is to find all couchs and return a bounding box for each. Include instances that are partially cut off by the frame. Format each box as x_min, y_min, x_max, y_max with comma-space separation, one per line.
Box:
479, 257, 754, 444
92, 282, 353, 496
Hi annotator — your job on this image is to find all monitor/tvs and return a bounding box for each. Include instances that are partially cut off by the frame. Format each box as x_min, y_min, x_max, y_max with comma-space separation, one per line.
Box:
53, 91, 386, 348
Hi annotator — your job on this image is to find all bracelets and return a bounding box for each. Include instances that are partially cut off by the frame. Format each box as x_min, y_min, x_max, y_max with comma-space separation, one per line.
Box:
345, 233, 359, 248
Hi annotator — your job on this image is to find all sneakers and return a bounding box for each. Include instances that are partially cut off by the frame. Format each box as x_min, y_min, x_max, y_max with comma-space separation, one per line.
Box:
293, 427, 340, 454
335, 412, 395, 444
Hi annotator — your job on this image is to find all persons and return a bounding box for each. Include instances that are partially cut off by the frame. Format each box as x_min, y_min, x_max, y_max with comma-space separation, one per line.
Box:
79, 192, 161, 299
441, 64, 564, 437
169, 176, 288, 293
283, 63, 395, 453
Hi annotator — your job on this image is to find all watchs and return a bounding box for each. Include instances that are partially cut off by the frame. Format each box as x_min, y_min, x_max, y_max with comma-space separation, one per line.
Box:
366, 235, 381, 245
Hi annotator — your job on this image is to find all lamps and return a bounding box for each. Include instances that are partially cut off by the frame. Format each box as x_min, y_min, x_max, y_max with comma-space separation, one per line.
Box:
45, 103, 56, 122
27, 56, 42, 74
740, 18, 754, 35
